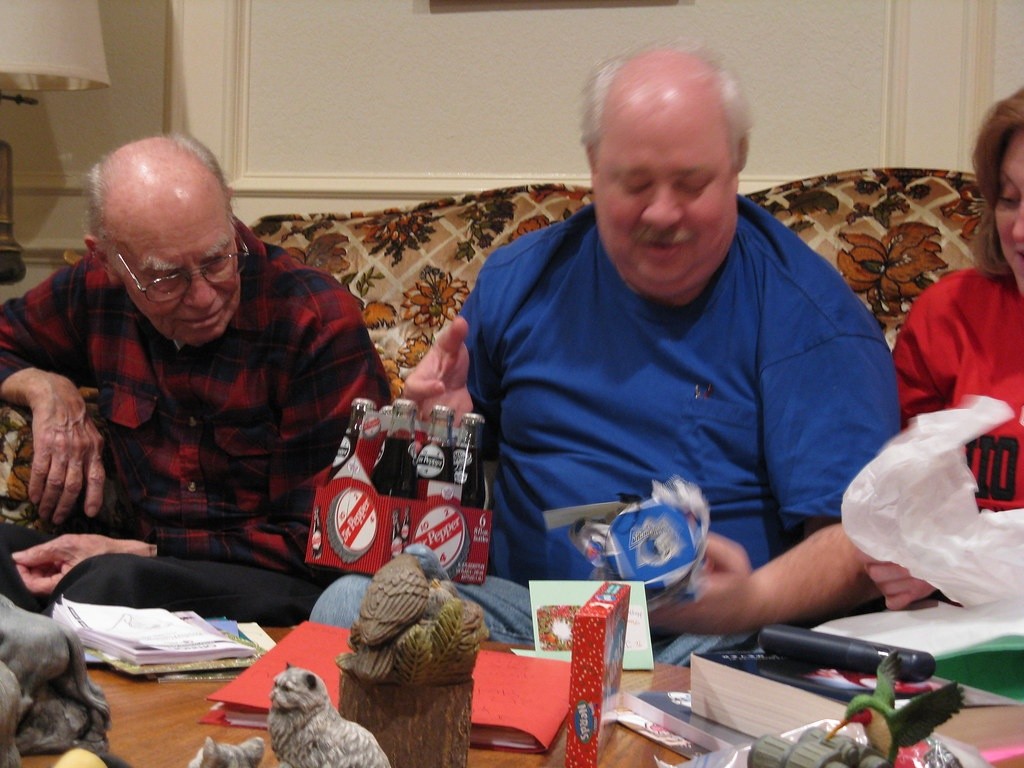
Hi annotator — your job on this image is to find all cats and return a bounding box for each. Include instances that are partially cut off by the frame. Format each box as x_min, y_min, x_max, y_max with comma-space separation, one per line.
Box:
185, 735, 266, 768
264, 660, 392, 767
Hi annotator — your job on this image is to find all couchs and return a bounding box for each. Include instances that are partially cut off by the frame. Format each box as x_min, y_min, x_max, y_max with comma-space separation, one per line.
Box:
0, 166, 1024, 638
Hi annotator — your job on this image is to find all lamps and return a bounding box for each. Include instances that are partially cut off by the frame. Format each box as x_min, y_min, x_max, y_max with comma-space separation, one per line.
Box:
0, 0, 110, 284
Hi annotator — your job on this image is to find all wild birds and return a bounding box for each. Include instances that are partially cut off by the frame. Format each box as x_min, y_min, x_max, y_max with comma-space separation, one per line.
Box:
341, 542, 475, 682
822, 649, 967, 768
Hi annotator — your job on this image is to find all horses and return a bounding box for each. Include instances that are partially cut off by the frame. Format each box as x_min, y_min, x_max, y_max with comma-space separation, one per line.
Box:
0, 591, 111, 768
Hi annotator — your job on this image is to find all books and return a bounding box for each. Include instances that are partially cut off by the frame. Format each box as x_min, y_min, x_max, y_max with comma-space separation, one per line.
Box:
620, 648, 945, 760
45, 591, 278, 683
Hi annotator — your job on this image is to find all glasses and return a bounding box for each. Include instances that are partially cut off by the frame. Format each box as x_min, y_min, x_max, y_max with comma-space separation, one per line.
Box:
118, 216, 252, 303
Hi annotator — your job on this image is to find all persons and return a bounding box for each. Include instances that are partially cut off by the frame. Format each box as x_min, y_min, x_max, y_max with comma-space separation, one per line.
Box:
865, 90, 1023, 612
308, 44, 902, 665
0, 129, 391, 628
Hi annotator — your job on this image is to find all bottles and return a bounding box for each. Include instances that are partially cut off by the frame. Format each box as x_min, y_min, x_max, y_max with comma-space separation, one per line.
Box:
321, 397, 487, 511
389, 506, 404, 560
399, 502, 413, 544
310, 507, 323, 559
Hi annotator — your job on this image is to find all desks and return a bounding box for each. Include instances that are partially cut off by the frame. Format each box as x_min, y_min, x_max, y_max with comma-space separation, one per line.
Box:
0, 625, 1024, 768
